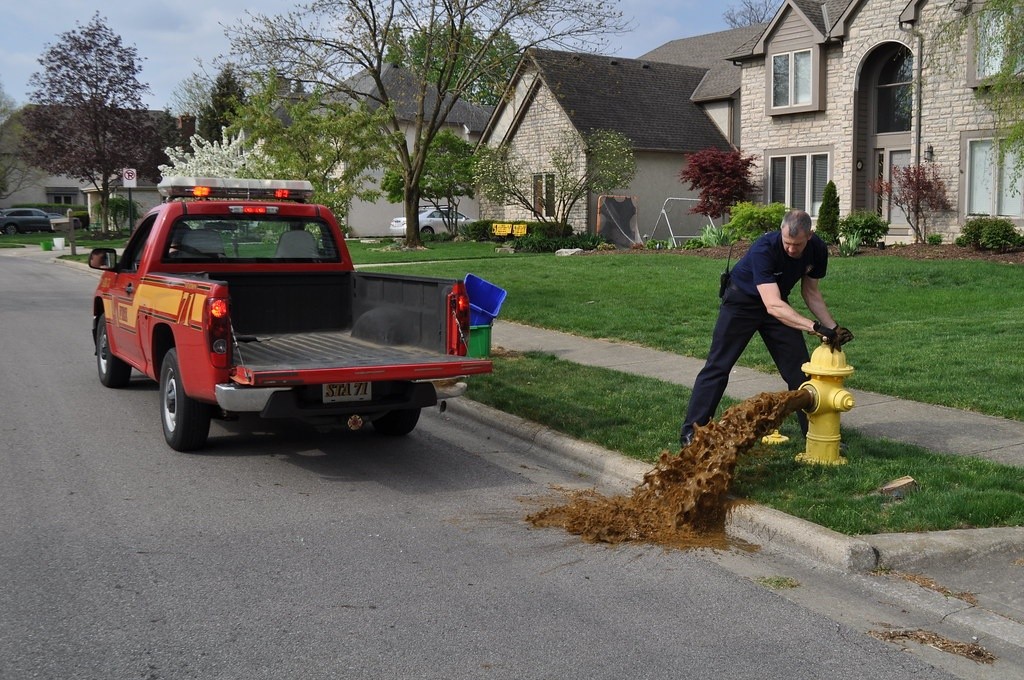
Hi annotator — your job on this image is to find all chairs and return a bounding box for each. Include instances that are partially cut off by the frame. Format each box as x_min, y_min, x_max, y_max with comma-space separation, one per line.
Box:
176, 229, 226, 258
275, 230, 321, 258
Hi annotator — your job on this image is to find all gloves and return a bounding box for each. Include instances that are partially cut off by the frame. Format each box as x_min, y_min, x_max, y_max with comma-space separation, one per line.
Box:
808, 320, 853, 353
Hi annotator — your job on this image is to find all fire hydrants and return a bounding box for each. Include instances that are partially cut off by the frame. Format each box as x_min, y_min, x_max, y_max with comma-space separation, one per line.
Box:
794, 336, 855, 466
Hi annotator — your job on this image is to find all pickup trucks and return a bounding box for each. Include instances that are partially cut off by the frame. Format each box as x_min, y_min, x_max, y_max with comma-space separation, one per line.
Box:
88, 176, 495, 452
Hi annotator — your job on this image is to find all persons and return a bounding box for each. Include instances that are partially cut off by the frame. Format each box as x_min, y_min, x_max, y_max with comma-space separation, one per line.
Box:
91, 251, 108, 268
169, 223, 192, 253
679, 210, 855, 451
290, 220, 306, 231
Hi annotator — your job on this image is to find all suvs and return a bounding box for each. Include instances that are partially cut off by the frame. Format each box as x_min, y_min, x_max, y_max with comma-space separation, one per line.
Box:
0, 209, 58, 235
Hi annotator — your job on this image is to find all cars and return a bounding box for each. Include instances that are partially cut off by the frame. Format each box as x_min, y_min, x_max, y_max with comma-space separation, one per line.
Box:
389, 209, 478, 237
47, 212, 82, 230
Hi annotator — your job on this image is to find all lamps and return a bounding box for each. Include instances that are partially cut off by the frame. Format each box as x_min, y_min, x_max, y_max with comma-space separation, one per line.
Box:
924, 141, 933, 161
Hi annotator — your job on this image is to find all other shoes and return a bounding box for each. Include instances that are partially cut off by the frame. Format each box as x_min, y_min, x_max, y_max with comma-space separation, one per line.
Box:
679, 435, 696, 448
